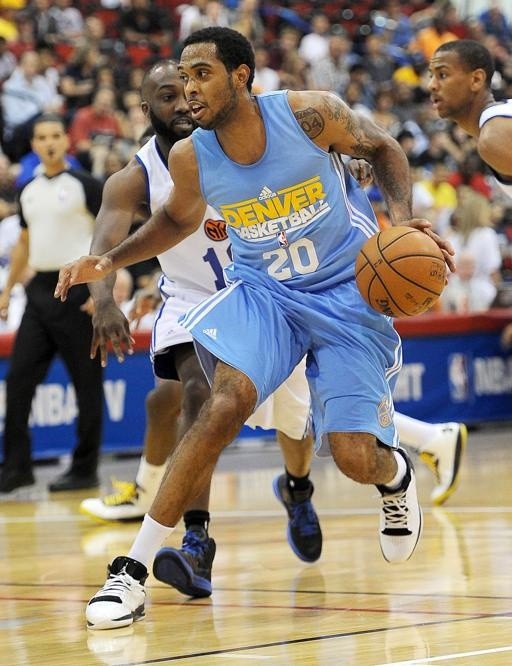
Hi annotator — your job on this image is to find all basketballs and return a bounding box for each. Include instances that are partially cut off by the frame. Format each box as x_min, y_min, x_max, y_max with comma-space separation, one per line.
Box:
354, 225, 447, 318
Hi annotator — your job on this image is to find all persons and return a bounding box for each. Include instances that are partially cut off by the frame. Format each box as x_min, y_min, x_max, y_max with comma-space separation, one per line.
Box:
82, 60, 374, 598
52, 25, 457, 634
79, 126, 468, 524
0, 113, 107, 505
2, 1, 512, 348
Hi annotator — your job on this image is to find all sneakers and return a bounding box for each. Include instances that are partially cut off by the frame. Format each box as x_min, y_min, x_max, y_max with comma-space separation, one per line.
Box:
272, 474, 322, 562
419, 421, 468, 504
0, 470, 28, 490
85, 557, 149, 630
153, 530, 216, 597
376, 446, 424, 563
80, 479, 153, 524
49, 475, 98, 490
